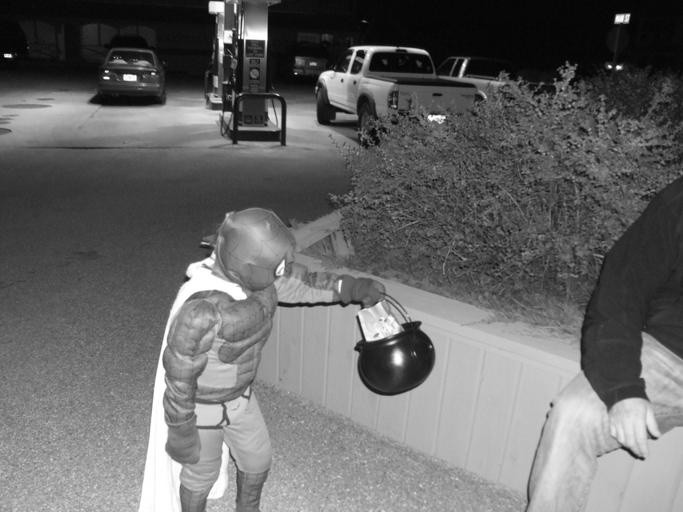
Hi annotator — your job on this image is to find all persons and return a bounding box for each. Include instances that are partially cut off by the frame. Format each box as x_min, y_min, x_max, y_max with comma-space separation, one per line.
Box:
161, 206, 386, 511
522, 174, 682, 512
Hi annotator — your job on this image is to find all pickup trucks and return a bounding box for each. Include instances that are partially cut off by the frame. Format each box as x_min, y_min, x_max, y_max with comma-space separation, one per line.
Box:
270, 41, 327, 79
313, 44, 476, 146
434, 56, 542, 105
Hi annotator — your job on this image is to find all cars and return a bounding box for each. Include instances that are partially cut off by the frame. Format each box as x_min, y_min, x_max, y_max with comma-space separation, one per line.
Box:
96, 47, 167, 105
0, 22, 30, 66
